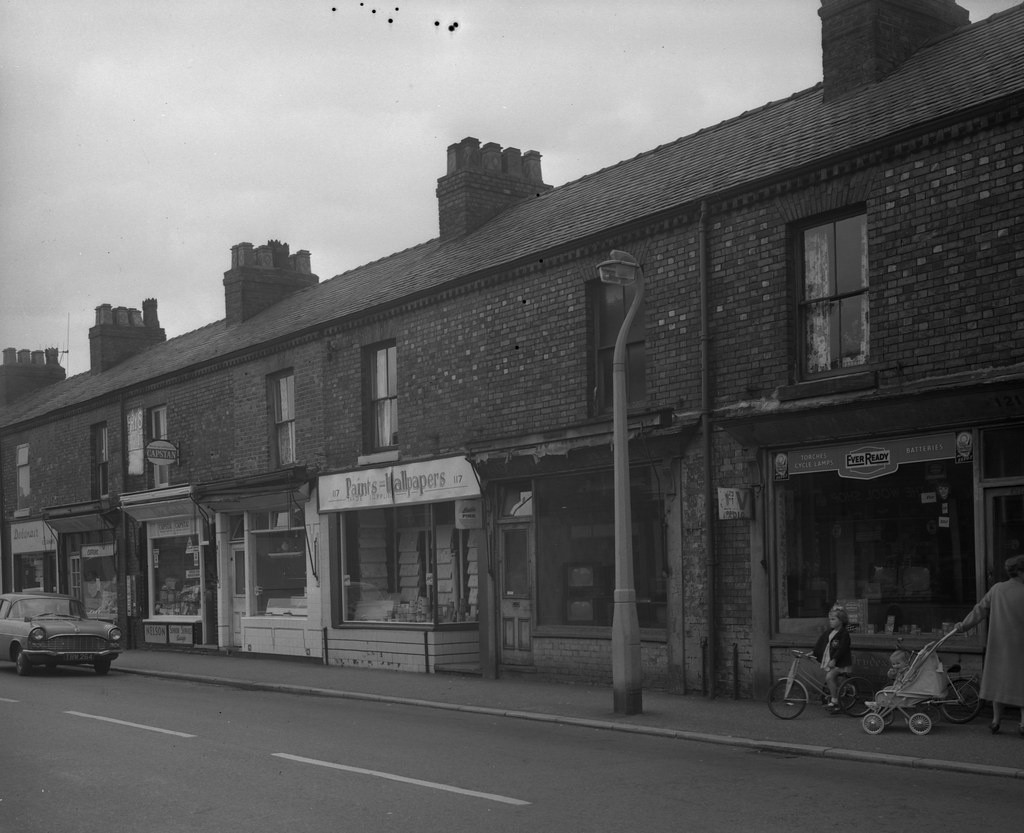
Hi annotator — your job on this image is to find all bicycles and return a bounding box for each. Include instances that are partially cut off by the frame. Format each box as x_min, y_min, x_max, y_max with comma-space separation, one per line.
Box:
767, 649, 877, 721
877, 634, 986, 724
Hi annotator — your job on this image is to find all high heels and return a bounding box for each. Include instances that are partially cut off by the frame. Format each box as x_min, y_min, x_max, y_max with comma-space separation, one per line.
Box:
989, 723, 1000, 735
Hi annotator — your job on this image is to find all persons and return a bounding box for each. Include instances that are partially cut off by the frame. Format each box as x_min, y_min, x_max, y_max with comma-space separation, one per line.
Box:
864, 651, 911, 711
954, 555, 1024, 738
804, 608, 853, 715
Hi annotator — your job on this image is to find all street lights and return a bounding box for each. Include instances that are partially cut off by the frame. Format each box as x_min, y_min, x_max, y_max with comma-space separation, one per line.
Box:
596, 248, 643, 717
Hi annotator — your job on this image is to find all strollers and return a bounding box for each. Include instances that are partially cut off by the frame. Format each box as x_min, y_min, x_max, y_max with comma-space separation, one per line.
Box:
861, 627, 965, 736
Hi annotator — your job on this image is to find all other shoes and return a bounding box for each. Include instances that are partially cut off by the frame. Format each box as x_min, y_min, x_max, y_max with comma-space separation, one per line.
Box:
865, 701, 878, 711
823, 702, 840, 708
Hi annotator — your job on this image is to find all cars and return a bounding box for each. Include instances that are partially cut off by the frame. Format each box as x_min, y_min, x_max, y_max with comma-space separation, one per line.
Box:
0, 590, 124, 678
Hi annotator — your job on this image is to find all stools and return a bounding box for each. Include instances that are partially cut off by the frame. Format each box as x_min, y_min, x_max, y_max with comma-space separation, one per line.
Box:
635, 596, 654, 629
